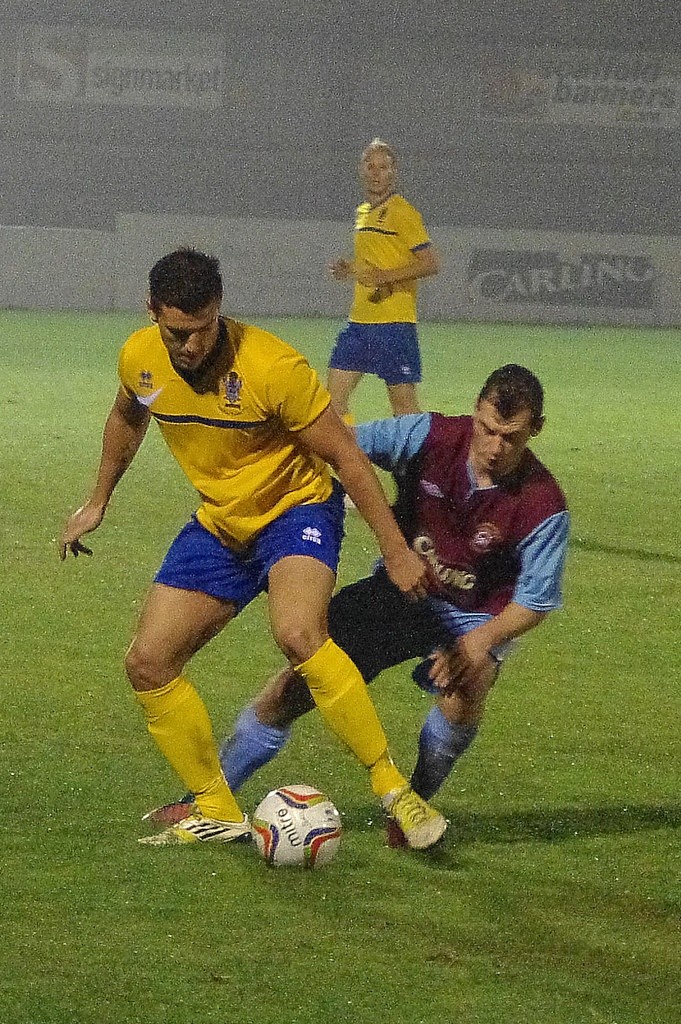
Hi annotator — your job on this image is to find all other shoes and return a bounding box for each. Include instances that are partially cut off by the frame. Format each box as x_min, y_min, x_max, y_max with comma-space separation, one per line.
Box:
387, 816, 408, 848
141, 800, 193, 825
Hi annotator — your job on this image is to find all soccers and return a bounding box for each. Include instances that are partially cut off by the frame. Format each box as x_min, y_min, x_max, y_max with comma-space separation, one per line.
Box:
251, 785, 342, 870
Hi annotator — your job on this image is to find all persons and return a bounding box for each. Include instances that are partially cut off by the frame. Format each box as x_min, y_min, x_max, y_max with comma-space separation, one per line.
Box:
57, 248, 448, 851
135, 361, 572, 846
325, 141, 440, 426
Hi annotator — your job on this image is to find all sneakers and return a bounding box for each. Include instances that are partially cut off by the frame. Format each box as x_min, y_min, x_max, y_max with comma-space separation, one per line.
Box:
137, 805, 252, 848
381, 781, 448, 851
